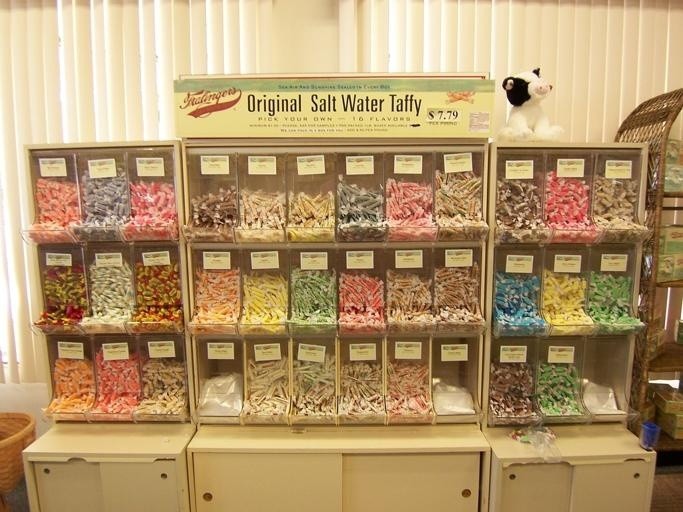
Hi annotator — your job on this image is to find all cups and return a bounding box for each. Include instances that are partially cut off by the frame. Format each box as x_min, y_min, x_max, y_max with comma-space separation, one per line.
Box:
639, 422, 661, 451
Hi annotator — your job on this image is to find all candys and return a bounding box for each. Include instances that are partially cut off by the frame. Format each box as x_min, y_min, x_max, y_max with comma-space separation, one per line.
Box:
29, 169, 643, 444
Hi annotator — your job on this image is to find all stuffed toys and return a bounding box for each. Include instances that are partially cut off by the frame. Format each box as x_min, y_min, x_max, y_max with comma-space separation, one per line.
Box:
491, 68, 566, 143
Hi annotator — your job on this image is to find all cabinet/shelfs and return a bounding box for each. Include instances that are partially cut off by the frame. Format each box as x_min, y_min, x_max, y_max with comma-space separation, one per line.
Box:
480, 141, 657, 512
615, 88, 683, 453
181, 137, 491, 512
21, 139, 197, 512
